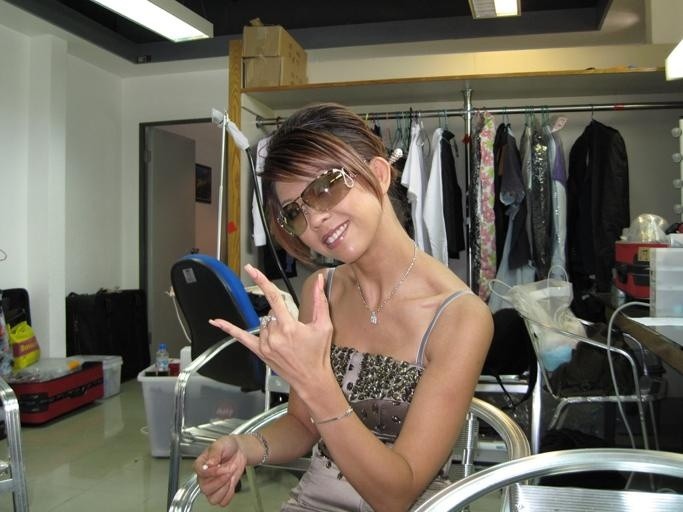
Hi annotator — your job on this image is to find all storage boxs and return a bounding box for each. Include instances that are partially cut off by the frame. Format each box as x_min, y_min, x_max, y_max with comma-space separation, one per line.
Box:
242, 55, 307, 88
132, 355, 265, 461
61, 354, 124, 400
240, 24, 306, 75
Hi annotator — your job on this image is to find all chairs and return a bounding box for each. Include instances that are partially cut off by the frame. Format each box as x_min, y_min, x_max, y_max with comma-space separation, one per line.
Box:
163, 382, 532, 510
411, 430, 682, 512
514, 304, 668, 495
168, 253, 290, 496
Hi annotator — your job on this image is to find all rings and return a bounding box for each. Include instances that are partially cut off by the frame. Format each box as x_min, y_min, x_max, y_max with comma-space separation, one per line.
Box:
258, 316, 276, 329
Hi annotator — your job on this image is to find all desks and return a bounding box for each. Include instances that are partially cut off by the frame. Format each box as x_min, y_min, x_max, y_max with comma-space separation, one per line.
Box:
595, 302, 681, 447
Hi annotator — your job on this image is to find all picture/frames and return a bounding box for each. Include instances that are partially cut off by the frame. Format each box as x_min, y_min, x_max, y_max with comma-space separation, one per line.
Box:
192, 162, 212, 206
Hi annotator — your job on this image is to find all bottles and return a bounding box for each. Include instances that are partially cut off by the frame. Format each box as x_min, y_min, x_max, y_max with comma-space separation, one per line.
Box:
155, 343, 169, 376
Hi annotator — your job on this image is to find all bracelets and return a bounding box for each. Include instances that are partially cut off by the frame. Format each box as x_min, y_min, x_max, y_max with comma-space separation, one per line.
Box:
309, 405, 354, 425
249, 431, 271, 467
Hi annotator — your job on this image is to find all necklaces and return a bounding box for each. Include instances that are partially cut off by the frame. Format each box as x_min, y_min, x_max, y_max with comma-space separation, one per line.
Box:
356, 240, 417, 326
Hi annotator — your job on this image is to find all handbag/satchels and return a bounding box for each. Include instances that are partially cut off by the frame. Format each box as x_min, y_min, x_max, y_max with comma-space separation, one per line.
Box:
488, 264, 589, 373
5, 320, 41, 370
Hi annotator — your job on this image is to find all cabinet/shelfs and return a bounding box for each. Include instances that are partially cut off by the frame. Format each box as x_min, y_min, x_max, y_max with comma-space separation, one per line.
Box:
66, 289, 150, 383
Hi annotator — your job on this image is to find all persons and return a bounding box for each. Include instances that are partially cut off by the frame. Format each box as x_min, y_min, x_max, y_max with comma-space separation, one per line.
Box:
192, 103, 494, 512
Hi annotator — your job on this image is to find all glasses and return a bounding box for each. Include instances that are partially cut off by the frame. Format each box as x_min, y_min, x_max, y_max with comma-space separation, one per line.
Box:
275, 158, 367, 239
